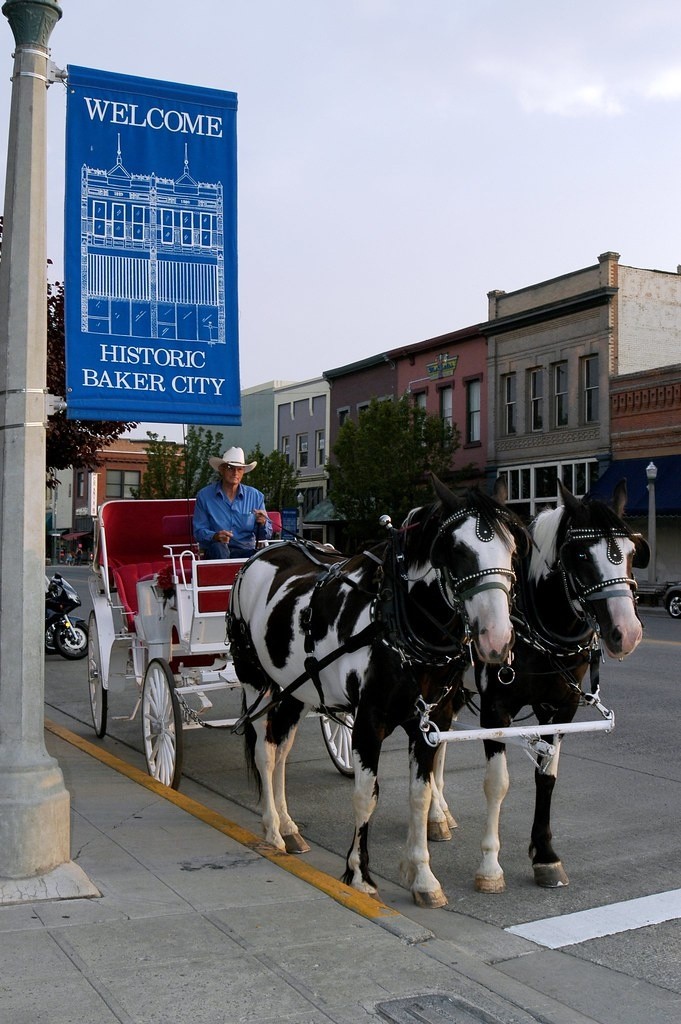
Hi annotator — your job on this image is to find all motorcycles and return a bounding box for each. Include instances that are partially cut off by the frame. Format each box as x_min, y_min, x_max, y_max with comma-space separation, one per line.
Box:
45, 572, 89, 660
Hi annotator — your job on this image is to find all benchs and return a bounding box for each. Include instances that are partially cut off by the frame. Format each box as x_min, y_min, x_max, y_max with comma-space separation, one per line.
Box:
97, 499, 282, 633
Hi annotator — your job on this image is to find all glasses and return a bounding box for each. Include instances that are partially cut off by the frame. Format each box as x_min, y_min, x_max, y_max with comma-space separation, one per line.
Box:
222, 465, 244, 472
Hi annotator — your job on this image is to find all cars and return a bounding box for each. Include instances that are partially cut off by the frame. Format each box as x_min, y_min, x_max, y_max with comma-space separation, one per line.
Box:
662, 586, 681, 619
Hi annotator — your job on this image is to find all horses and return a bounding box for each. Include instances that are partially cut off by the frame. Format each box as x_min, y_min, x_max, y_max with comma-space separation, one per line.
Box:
427, 477, 642, 889
228, 473, 515, 910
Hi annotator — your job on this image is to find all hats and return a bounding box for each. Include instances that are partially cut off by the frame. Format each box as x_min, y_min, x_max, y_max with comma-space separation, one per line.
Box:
209, 447, 258, 473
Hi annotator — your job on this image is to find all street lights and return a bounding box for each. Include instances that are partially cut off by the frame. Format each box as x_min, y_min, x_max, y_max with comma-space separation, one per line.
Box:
645, 461, 657, 586
297, 493, 304, 538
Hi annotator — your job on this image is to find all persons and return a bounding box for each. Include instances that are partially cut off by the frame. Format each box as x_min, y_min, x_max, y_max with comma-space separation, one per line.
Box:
192, 447, 274, 559
66, 546, 83, 566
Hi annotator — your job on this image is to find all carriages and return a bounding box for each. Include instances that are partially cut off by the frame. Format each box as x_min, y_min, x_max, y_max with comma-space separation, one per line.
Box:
88, 471, 650, 910
